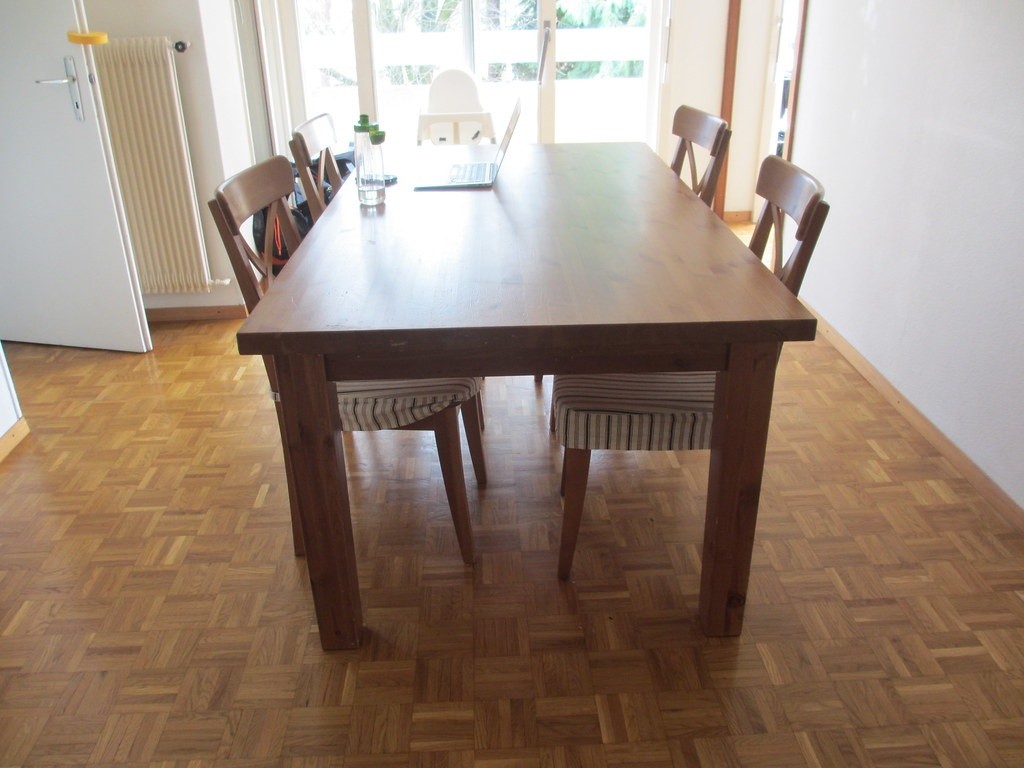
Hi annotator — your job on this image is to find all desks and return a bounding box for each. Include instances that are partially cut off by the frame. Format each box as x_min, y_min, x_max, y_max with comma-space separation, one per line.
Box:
241, 141, 819, 652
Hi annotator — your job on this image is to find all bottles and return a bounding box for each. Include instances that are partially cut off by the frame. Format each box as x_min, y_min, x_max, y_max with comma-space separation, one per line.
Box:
354, 114, 385, 205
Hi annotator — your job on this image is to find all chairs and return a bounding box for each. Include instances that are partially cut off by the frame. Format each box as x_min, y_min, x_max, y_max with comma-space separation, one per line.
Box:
418, 68, 497, 142
532, 102, 729, 393
207, 156, 488, 566
553, 153, 831, 585
290, 112, 489, 490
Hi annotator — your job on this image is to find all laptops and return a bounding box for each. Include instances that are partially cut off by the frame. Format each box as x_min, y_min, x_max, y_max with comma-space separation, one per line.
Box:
415, 98, 523, 189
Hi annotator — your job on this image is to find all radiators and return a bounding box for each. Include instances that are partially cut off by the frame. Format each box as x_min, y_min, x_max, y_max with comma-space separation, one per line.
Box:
90, 37, 230, 296
